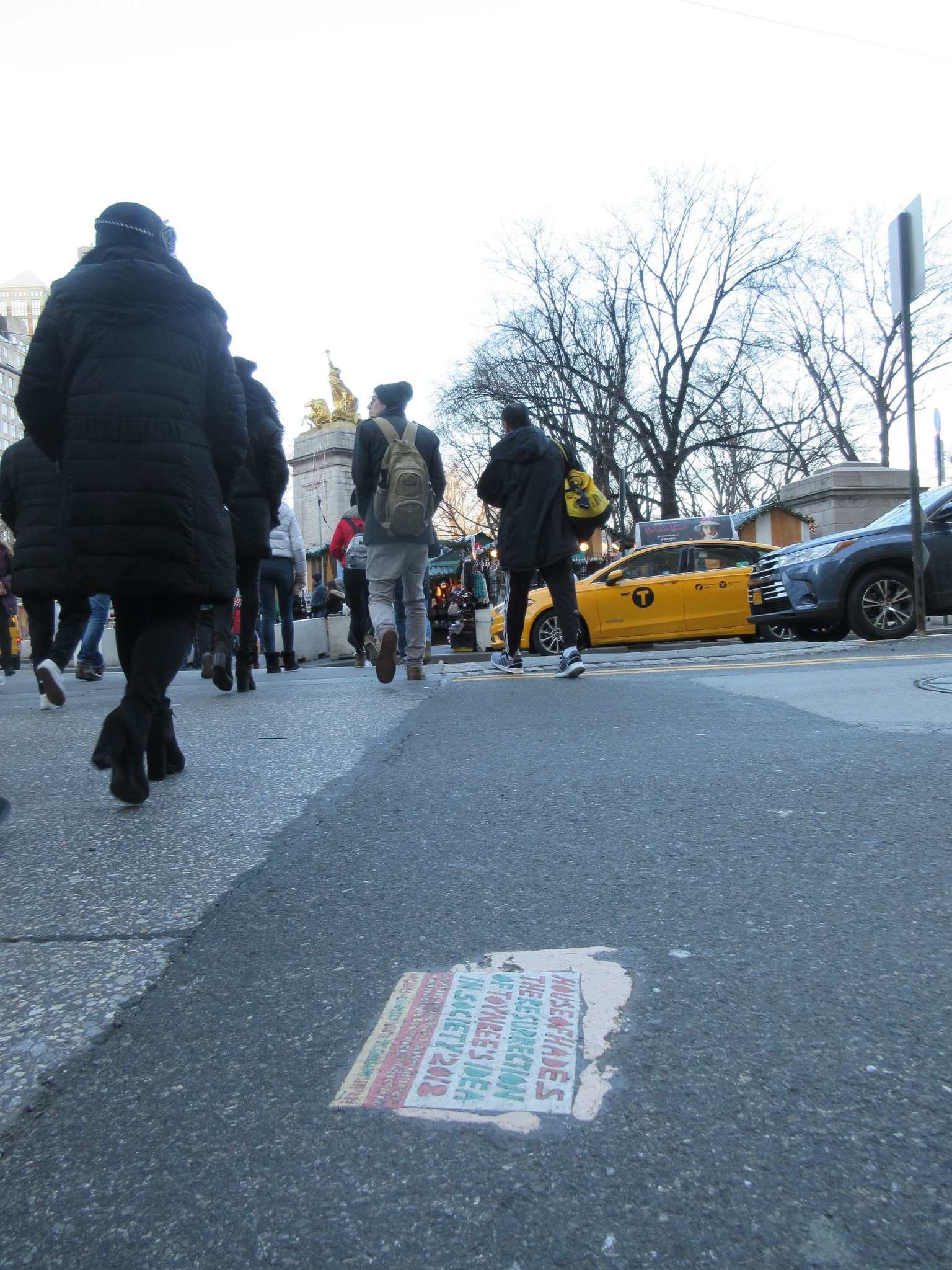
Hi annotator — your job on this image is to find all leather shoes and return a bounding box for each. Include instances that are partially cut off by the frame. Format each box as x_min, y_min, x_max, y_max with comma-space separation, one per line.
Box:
75, 661, 104, 681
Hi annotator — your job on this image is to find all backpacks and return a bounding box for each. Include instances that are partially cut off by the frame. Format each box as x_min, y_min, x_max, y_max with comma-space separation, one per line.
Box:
371, 417, 436, 540
344, 517, 369, 570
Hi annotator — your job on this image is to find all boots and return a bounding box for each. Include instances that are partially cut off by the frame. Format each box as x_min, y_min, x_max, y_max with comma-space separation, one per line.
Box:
263, 651, 282, 673
363, 634, 380, 666
280, 649, 300, 671
353, 652, 365, 668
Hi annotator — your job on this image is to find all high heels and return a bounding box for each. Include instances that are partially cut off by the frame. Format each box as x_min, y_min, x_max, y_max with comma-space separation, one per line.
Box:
235, 660, 256, 693
211, 642, 234, 691
91, 695, 149, 805
144, 707, 186, 783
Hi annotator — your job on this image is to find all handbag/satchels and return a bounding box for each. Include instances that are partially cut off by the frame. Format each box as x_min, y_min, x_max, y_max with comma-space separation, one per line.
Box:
564, 468, 614, 538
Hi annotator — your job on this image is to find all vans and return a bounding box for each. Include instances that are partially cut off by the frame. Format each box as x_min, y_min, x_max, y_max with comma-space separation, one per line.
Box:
0, 615, 21, 669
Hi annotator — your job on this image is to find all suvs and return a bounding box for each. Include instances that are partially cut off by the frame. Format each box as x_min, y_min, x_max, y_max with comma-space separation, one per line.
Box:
744, 480, 952, 641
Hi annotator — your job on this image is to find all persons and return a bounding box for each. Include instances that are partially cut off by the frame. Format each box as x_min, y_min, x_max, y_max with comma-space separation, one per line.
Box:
476, 401, 595, 677
329, 486, 384, 668
351, 380, 447, 684
694, 517, 727, 541
571, 547, 746, 650
256, 497, 308, 674
0, 424, 91, 711
71, 593, 111, 680
393, 561, 432, 665
12, 200, 258, 804
211, 352, 291, 693
177, 563, 348, 674
0, 539, 19, 678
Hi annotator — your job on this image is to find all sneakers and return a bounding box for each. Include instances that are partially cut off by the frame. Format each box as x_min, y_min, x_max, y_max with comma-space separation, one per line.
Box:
200, 651, 214, 679
405, 666, 426, 680
490, 651, 524, 675
554, 650, 586, 678
376, 628, 398, 684
37, 658, 67, 710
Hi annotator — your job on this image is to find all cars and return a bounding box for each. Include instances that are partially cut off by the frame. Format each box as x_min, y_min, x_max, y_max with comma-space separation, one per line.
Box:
487, 537, 782, 658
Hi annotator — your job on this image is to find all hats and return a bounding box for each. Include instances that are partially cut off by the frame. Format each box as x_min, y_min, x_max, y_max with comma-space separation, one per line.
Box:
93, 202, 177, 254
693, 517, 725, 533
312, 572, 322, 581
373, 381, 414, 411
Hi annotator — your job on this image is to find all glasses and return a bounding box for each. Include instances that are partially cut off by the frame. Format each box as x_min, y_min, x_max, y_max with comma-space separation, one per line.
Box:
370, 396, 377, 405
